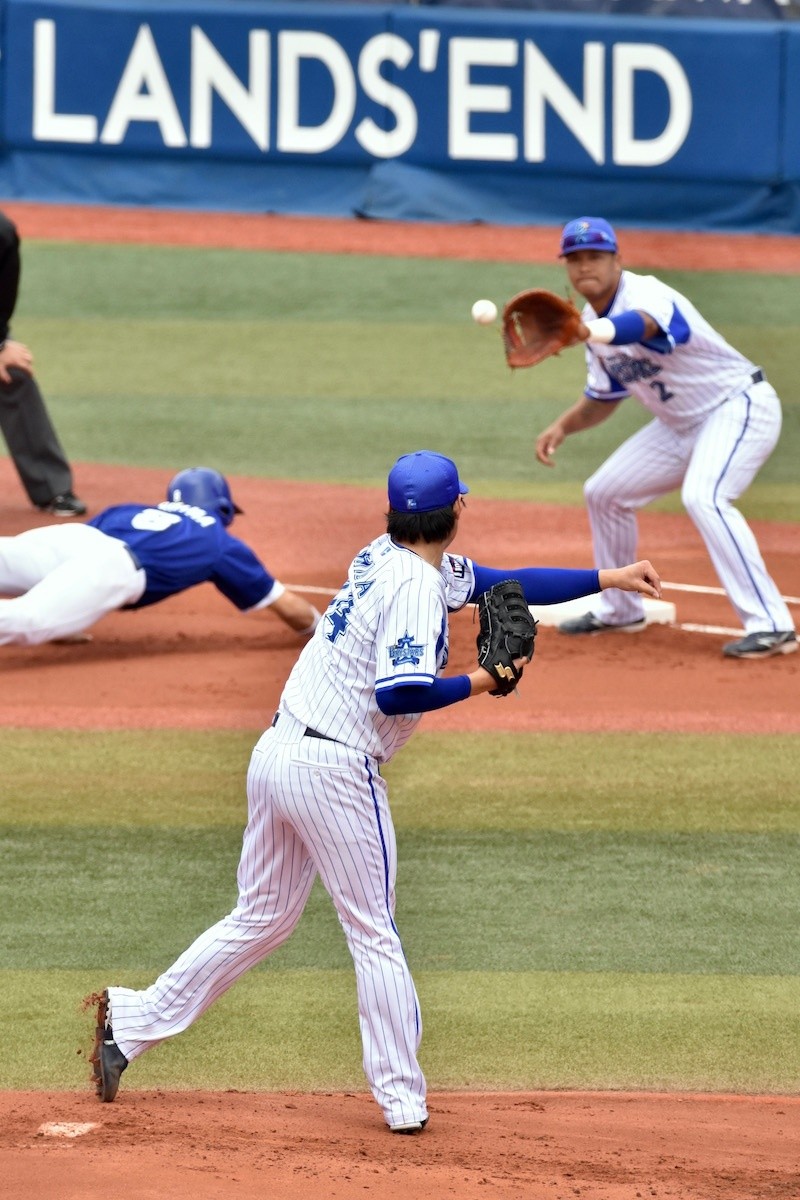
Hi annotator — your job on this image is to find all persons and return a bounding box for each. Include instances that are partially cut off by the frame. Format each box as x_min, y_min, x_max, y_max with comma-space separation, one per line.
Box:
501, 215, 799, 663
0, 462, 324, 653
1, 207, 90, 519
83, 449, 664, 1136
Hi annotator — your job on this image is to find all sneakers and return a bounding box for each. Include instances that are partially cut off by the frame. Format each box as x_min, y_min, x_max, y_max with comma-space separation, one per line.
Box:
724, 625, 799, 661
559, 613, 649, 634
41, 490, 86, 519
89, 990, 130, 1101
389, 1118, 428, 1132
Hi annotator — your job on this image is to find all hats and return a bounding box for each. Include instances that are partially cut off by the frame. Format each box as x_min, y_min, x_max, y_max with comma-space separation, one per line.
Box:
557, 214, 615, 257
388, 450, 470, 512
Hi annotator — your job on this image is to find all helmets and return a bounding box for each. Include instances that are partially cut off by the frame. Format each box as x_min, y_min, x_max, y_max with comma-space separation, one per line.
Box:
167, 468, 244, 527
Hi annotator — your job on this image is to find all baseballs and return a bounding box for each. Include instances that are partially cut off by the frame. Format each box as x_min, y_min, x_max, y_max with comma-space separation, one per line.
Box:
471, 299, 498, 325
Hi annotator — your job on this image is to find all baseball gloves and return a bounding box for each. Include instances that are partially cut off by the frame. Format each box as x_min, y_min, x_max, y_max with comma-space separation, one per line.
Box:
499, 283, 582, 375
473, 578, 543, 701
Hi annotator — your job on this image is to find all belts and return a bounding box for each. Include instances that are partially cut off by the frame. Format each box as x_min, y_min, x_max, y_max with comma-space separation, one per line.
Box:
124, 546, 144, 572
273, 712, 329, 740
752, 370, 764, 385
